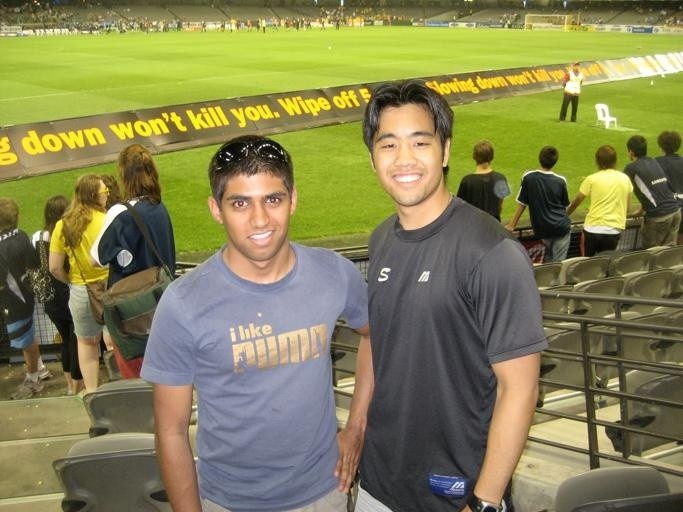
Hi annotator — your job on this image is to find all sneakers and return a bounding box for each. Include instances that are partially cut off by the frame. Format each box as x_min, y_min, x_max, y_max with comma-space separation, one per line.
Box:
10, 366, 51, 400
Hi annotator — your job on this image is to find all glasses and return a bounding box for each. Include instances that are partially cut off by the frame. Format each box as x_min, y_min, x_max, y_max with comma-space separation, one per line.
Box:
97, 187, 109, 196
212, 138, 288, 172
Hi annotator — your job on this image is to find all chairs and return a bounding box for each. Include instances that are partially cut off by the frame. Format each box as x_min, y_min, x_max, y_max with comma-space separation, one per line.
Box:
83, 377, 154, 438
533, 245, 683, 512
52, 432, 173, 512
595, 103, 617, 129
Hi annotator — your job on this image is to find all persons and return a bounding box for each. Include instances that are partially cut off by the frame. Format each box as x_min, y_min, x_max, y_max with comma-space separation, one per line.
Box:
351, 1, 414, 25
0, 1, 190, 34
653, 131, 683, 247
452, 138, 511, 224
559, 61, 584, 122
544, 0, 683, 26
91, 143, 177, 383
506, 145, 569, 265
619, 136, 680, 250
566, 144, 634, 259
2, 174, 124, 399
451, 9, 519, 28
138, 135, 374, 512
352, 79, 549, 512
199, 1, 349, 35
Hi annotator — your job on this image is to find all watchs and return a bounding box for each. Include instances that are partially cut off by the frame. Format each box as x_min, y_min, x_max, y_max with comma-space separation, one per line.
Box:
466, 488, 507, 512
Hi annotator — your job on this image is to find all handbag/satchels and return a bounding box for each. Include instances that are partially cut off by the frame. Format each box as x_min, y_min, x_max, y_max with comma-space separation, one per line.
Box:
26, 266, 55, 304
86, 281, 108, 326
103, 264, 174, 362
